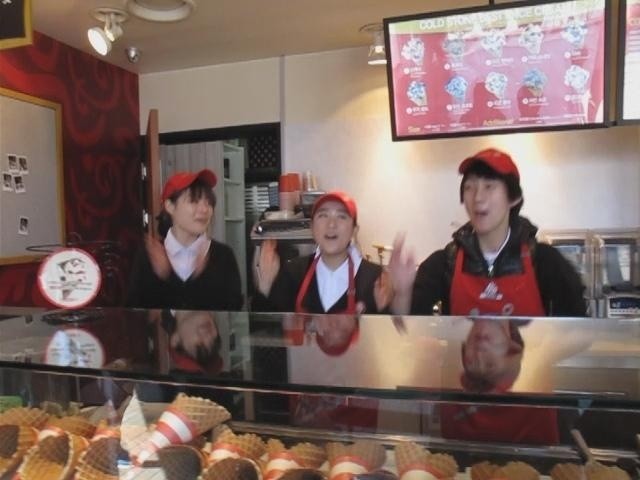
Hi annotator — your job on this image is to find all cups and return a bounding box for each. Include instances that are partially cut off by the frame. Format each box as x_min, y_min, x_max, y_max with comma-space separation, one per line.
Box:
244, 171, 332, 220
584, 296, 605, 319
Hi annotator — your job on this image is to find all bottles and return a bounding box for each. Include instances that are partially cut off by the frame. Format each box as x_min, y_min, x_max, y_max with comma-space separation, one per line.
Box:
223, 159, 230, 179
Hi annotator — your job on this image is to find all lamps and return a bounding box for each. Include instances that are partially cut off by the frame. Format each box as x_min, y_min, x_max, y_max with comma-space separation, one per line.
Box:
358, 23, 389, 66
87, 7, 129, 58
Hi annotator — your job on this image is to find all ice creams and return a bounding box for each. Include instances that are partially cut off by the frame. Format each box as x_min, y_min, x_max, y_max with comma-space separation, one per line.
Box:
55, 258, 86, 299
401, 17, 591, 107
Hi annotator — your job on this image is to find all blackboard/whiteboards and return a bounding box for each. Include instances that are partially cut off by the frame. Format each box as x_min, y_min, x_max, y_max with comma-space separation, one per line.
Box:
2, 90, 64, 265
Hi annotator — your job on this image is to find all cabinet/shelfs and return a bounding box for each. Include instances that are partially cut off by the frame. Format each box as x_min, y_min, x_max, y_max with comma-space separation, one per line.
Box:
211, 311, 254, 424
0, 306, 640, 480
160, 141, 249, 311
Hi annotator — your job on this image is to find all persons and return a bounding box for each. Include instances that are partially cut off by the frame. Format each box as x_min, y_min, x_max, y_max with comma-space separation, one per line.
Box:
459, 316, 533, 394
251, 190, 391, 313
282, 310, 411, 356
147, 310, 224, 377
387, 148, 587, 317
124, 168, 243, 310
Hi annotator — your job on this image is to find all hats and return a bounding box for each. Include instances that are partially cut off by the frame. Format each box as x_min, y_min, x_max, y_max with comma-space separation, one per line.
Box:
312, 191, 358, 223
460, 364, 521, 395
314, 326, 360, 358
170, 346, 225, 379
458, 148, 520, 184
160, 168, 217, 203
